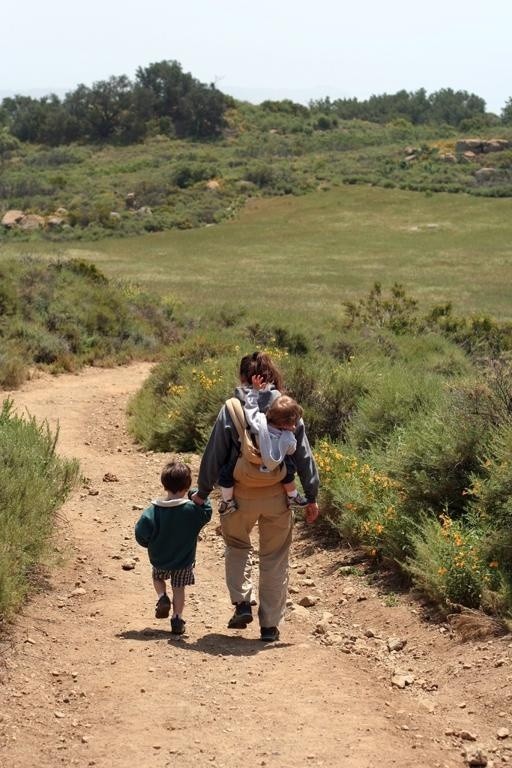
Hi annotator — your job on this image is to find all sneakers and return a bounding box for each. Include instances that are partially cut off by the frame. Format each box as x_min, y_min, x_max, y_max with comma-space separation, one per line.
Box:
228, 604, 253, 629
287, 494, 310, 509
218, 500, 238, 515
261, 628, 281, 641
155, 596, 185, 633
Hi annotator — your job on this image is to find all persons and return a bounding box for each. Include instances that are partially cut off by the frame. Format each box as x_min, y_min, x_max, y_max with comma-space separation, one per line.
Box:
191, 352, 319, 641
135, 461, 212, 635
